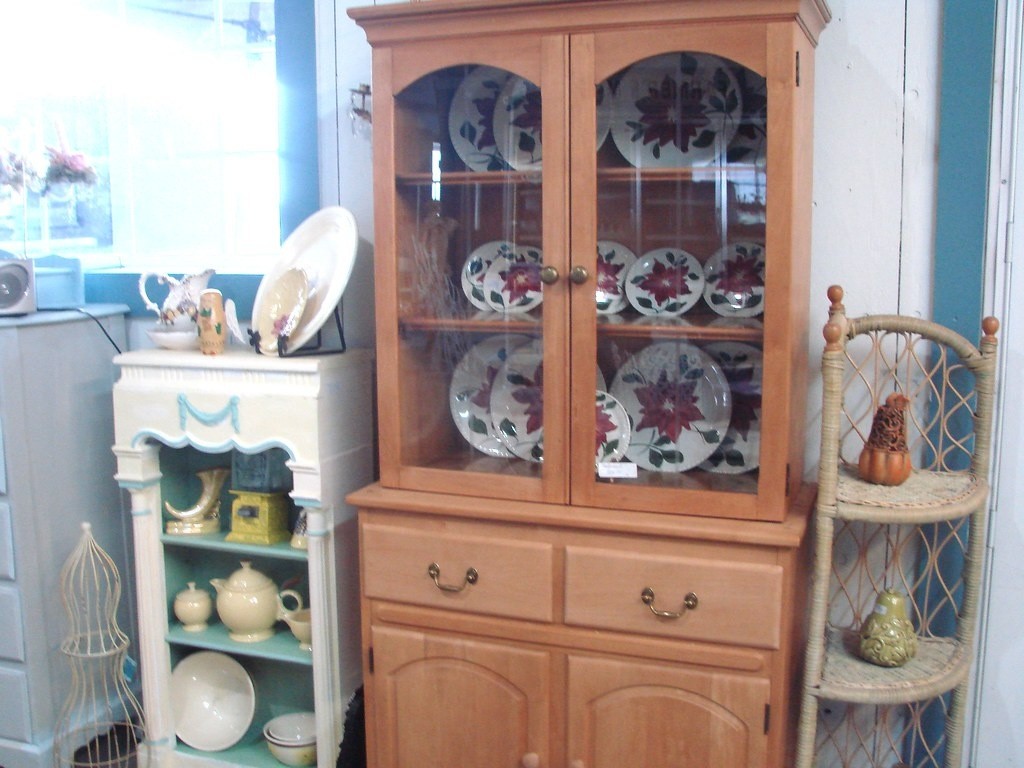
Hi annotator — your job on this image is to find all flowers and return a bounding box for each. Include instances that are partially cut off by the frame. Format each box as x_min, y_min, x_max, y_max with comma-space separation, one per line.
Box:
0, 148, 95, 194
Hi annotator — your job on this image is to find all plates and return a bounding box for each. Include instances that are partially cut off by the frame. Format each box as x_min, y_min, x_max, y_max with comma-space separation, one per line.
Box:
170, 649, 255, 752
699, 339, 765, 476
461, 241, 518, 311
450, 333, 532, 459
254, 270, 308, 350
624, 247, 704, 319
250, 207, 357, 355
489, 337, 607, 466
593, 239, 641, 315
724, 57, 766, 164
610, 339, 732, 474
610, 50, 742, 169
448, 63, 517, 172
493, 66, 614, 171
590, 388, 630, 467
702, 242, 765, 317
483, 245, 550, 314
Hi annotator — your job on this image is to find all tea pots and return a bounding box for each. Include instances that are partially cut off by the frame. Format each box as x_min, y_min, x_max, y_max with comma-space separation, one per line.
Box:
209, 560, 303, 642
174, 581, 212, 632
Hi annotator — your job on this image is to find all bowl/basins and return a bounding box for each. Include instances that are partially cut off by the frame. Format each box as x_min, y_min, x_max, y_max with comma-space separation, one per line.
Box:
263, 709, 318, 767
147, 330, 200, 349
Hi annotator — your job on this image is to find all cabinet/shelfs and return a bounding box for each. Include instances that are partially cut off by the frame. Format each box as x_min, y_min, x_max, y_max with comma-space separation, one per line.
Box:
794, 283, 999, 768
346, 480, 819, 768
110, 348, 376, 767
0, 303, 143, 768
348, 0, 832, 523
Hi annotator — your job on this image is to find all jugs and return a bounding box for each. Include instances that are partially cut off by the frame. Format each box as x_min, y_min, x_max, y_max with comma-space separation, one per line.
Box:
138, 268, 216, 330
